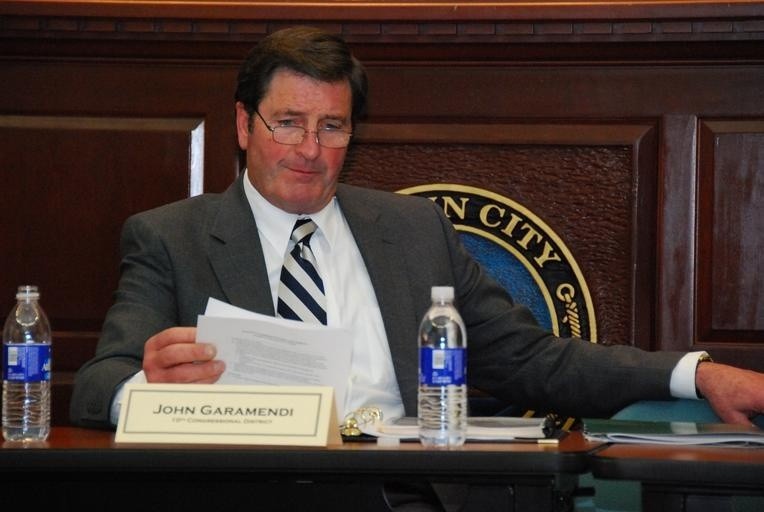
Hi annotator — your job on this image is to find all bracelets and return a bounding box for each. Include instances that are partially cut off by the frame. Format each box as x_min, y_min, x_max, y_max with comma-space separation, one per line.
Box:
695, 354, 713, 401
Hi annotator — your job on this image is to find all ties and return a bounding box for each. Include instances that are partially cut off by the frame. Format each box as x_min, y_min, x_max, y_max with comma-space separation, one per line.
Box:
277, 219, 327, 325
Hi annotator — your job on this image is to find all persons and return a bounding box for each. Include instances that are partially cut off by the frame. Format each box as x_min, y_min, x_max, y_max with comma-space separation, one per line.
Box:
65, 24, 764, 433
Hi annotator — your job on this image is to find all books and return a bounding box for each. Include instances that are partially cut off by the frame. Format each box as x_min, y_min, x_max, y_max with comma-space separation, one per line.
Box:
580, 418, 764, 449
372, 411, 561, 440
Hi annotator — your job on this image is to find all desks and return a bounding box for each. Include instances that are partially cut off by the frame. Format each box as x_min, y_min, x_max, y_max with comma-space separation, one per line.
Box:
0, 424, 764, 512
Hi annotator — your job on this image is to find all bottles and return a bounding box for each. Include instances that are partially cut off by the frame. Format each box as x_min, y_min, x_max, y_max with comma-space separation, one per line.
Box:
2, 285, 51, 443
416, 285, 469, 448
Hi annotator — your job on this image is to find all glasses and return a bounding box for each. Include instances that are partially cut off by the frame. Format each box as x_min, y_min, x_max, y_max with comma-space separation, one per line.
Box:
256, 110, 353, 148
